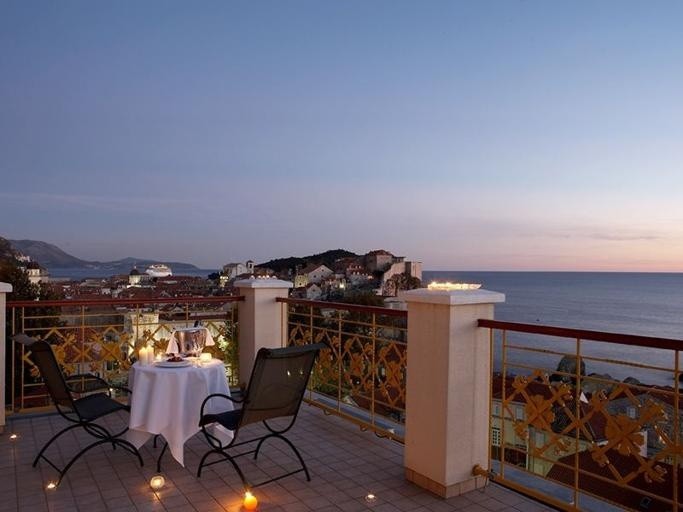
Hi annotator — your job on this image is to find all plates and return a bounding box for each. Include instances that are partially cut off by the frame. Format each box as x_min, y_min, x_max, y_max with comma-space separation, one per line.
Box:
151, 358, 190, 368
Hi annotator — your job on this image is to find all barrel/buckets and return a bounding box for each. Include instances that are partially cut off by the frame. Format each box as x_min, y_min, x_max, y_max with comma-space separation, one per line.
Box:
176, 329, 206, 354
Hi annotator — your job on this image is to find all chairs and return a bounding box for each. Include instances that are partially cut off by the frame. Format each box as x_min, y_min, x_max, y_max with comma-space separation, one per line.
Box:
26, 341, 144, 486
197, 344, 326, 494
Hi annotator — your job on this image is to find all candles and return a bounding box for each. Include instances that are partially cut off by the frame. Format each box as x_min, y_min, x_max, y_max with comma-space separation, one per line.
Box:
150, 475, 164, 490
243, 491, 257, 510
200, 353, 211, 363
138, 347, 148, 366
146, 345, 154, 362
